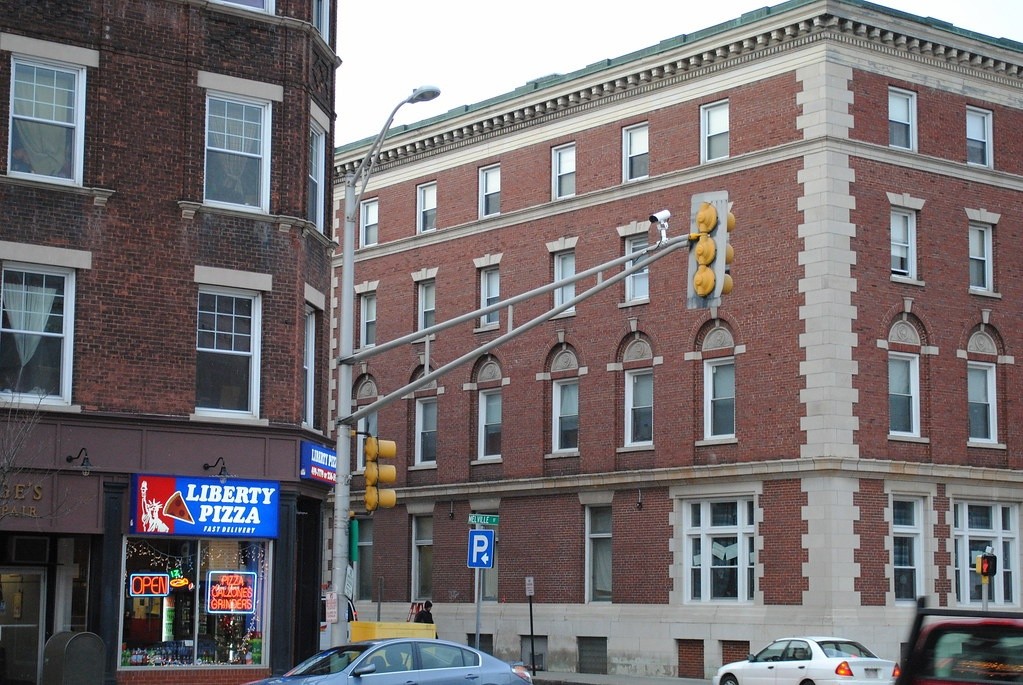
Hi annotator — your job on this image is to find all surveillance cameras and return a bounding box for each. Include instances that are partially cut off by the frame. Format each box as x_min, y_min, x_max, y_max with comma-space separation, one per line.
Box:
649, 209, 670, 223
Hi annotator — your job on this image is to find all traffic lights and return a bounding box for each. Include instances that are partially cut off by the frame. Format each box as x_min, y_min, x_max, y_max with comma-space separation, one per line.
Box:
975, 553, 997, 576
364, 436, 397, 509
688, 191, 739, 312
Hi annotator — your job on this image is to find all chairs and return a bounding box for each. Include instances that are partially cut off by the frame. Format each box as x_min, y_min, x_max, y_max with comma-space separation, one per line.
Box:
385, 648, 408, 672
451, 655, 469, 667
793, 648, 806, 659
371, 656, 386, 673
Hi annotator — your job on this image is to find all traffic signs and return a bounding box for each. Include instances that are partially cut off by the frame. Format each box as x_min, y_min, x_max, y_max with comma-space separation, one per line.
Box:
466, 529, 499, 570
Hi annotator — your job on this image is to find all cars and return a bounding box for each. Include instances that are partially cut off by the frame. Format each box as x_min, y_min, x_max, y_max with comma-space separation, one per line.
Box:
239, 637, 533, 685
710, 636, 900, 685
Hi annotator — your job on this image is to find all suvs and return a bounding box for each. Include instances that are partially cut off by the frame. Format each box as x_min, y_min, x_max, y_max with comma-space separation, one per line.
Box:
892, 597, 1023, 685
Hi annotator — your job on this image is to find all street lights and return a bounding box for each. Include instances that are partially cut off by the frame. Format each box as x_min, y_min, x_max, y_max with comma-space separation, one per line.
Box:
330, 84, 443, 670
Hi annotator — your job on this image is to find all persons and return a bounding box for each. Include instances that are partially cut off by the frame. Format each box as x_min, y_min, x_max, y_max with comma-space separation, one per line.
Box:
414, 600, 438, 638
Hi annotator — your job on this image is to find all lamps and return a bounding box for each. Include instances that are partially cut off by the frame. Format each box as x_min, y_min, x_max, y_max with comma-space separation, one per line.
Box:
203, 457, 239, 484
66, 448, 101, 476
